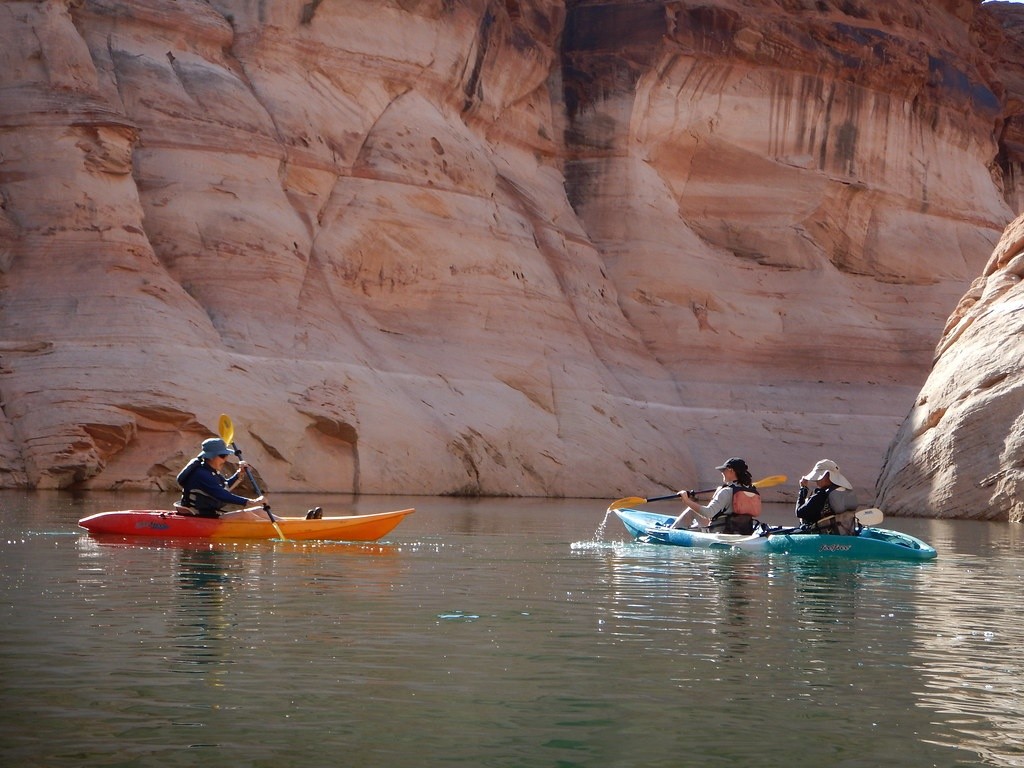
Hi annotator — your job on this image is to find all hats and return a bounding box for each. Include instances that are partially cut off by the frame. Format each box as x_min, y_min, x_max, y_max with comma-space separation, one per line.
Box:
714, 457, 748, 473
196, 438, 236, 459
802, 459, 854, 491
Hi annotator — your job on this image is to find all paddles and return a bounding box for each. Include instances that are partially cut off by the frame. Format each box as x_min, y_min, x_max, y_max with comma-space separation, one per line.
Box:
608, 474, 788, 512
733, 506, 885, 546
215, 413, 288, 542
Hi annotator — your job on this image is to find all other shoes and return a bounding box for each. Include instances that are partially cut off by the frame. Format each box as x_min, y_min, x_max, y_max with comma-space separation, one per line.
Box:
305, 507, 323, 520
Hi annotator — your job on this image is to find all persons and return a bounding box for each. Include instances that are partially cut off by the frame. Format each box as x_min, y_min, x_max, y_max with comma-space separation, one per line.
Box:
795, 457, 862, 536
671, 458, 760, 535
177, 437, 324, 520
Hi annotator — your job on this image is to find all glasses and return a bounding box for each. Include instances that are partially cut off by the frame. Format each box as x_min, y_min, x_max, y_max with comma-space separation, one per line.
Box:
218, 454, 228, 458
719, 469, 727, 472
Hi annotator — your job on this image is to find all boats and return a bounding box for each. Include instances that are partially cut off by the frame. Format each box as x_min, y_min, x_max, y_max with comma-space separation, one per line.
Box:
611, 507, 939, 560
77, 506, 417, 543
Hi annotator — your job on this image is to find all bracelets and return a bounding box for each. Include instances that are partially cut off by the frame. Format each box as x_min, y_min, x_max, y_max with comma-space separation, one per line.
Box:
799, 487, 810, 494
